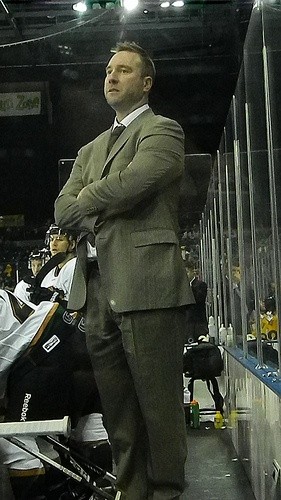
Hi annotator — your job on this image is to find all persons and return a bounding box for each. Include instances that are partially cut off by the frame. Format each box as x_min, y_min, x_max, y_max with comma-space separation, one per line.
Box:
55, 42, 196, 500
0, 210, 281, 500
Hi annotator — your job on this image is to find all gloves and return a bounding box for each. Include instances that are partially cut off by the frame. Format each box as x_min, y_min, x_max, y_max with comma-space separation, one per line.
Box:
26, 285, 53, 304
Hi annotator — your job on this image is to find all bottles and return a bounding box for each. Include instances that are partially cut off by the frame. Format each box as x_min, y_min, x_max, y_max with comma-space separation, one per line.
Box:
213, 410, 223, 429
189, 400, 200, 429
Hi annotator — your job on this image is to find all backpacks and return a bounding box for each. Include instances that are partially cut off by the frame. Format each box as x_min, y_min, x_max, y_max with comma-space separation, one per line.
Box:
180, 340, 224, 378
242, 339, 279, 367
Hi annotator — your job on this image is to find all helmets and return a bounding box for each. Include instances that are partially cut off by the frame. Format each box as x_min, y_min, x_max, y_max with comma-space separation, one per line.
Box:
28, 248, 51, 269
45, 223, 76, 245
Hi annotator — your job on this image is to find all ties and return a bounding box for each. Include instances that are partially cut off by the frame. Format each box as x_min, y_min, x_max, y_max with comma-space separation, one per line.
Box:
105, 125, 125, 158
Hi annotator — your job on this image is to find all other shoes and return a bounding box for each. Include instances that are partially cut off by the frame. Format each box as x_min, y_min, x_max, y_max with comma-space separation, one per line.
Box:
8, 456, 68, 499
65, 439, 113, 494
